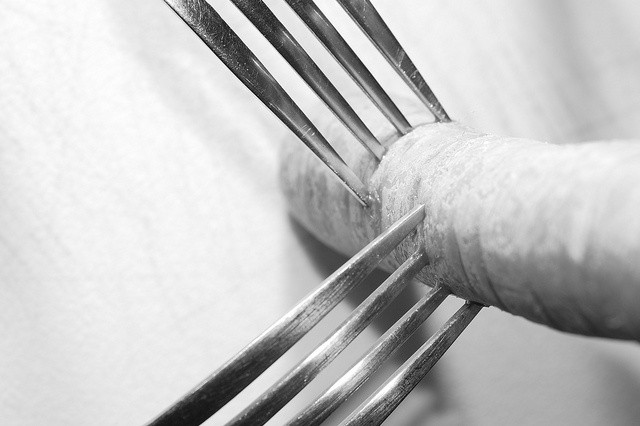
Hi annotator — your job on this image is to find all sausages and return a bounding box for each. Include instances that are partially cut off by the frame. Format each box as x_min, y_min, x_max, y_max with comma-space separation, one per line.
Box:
277, 103, 639, 339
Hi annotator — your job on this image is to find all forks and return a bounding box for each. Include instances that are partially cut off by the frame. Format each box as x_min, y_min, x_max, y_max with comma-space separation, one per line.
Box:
163, 0, 452, 217
142, 204, 483, 426
